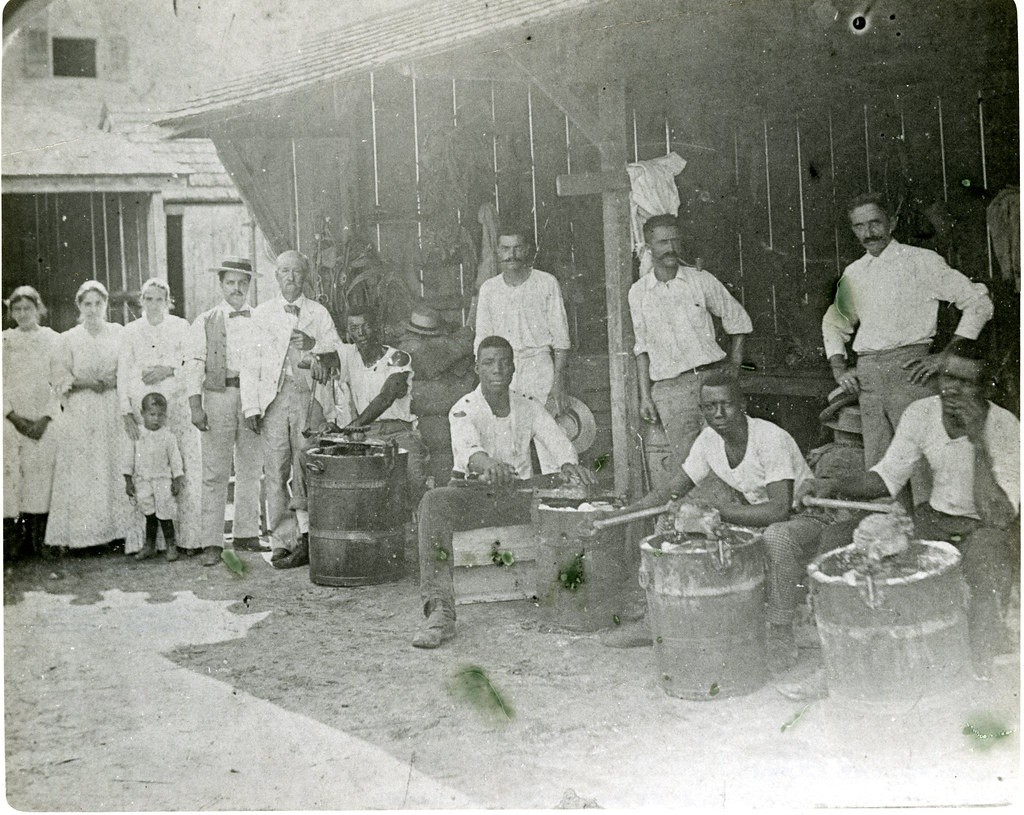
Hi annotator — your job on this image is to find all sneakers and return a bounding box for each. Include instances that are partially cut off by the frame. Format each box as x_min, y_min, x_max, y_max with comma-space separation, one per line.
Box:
411, 598, 457, 648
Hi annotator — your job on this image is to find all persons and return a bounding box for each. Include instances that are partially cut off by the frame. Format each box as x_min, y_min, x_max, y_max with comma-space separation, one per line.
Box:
579, 373, 831, 673
821, 187, 994, 518
2, 251, 425, 566
412, 335, 599, 648
472, 225, 570, 471
779, 341, 1021, 700
628, 213, 754, 505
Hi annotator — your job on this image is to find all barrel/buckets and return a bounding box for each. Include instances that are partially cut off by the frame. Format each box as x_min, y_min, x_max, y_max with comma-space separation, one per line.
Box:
530, 490, 627, 633
806, 538, 972, 732
306, 439, 408, 588
639, 525, 770, 702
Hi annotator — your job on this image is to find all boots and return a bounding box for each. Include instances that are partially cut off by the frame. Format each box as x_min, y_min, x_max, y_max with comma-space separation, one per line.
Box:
18, 513, 36, 548
3, 517, 27, 573
35, 513, 55, 561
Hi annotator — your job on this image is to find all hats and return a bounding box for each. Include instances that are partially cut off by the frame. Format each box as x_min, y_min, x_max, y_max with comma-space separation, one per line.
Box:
543, 394, 596, 454
208, 256, 264, 279
819, 387, 864, 436
403, 306, 442, 335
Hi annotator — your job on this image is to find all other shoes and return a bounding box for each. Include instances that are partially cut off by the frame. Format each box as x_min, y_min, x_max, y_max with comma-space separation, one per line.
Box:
201, 546, 223, 565
608, 618, 654, 647
767, 624, 799, 672
971, 645, 995, 682
166, 544, 179, 561
776, 669, 830, 701
273, 538, 309, 568
271, 546, 291, 561
135, 543, 157, 560
233, 536, 271, 552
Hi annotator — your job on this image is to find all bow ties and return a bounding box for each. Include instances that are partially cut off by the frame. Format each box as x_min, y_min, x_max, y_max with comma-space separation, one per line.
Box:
284, 305, 301, 317
229, 310, 252, 318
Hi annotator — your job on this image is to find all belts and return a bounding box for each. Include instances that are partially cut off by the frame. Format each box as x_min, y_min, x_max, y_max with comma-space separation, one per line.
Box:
224, 377, 241, 388
680, 360, 724, 375
